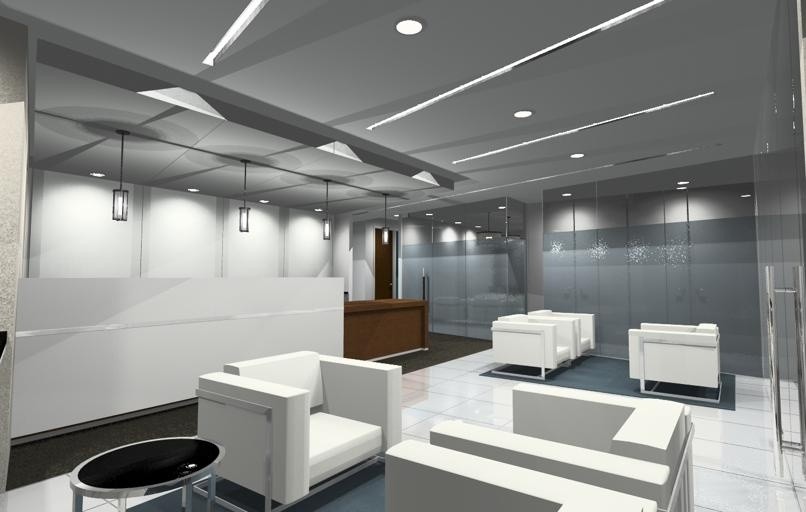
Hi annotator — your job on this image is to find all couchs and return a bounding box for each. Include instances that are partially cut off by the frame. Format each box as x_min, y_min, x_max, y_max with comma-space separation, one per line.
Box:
525, 309, 597, 368
385, 440, 660, 512
626, 322, 724, 404
489, 312, 574, 381
427, 379, 695, 512
196, 348, 404, 511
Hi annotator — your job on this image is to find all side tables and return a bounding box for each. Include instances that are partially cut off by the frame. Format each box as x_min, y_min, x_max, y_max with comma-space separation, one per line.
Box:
69, 436, 225, 511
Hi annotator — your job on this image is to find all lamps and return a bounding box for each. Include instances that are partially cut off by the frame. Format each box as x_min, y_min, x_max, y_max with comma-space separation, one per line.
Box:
237, 158, 250, 232
320, 178, 333, 241
381, 192, 390, 245
111, 129, 131, 222
